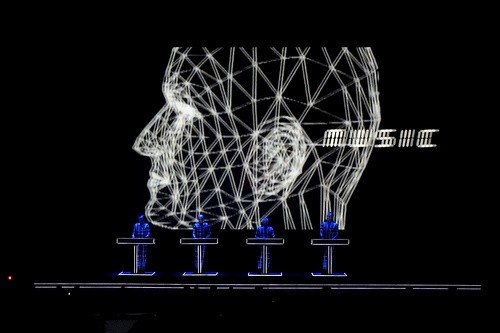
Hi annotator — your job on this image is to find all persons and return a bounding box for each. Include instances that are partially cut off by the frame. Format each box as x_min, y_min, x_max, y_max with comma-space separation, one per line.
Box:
320, 212, 338, 270
193, 215, 211, 268
255, 218, 275, 269
132, 215, 150, 271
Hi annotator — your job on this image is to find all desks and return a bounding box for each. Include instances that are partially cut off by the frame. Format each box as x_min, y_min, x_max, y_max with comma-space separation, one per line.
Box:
246, 238, 287, 278
116, 237, 156, 276
310, 238, 350, 279
179, 238, 220, 277
